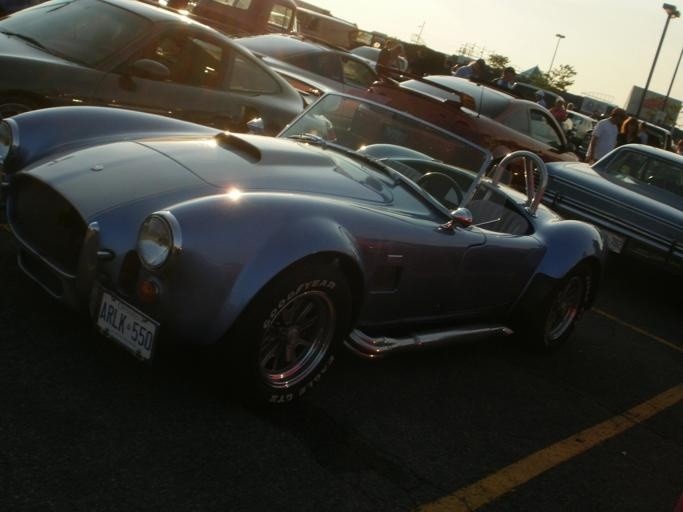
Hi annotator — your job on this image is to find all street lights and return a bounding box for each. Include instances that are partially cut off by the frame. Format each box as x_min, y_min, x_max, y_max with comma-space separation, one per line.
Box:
543, 31, 564, 79
547, 31, 566, 75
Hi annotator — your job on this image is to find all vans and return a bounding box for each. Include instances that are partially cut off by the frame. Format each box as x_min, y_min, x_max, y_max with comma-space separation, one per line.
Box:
507, 82, 562, 109
628, 117, 673, 150
179, 1, 298, 37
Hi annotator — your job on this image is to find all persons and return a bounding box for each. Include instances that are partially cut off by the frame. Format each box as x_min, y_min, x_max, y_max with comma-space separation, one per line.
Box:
676, 139, 683, 154
455, 59, 485, 82
536, 90, 546, 108
548, 100, 568, 122
617, 118, 642, 147
375, 40, 392, 74
638, 122, 648, 144
568, 103, 576, 110
586, 107, 625, 164
389, 44, 402, 77
490, 67, 517, 92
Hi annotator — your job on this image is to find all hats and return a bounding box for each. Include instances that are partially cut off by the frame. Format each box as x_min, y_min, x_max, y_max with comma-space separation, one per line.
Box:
505, 67, 518, 76
478, 59, 486, 69
536, 89, 545, 97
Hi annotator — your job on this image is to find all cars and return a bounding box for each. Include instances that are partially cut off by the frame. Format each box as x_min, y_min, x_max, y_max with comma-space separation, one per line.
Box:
562, 113, 597, 146
349, 45, 409, 75
231, 35, 381, 130
536, 143, 683, 275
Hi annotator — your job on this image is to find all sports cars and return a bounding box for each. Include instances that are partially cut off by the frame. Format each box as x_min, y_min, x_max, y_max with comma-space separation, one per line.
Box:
523, 144, 682, 285
1, 92, 604, 404
2, 1, 337, 141
350, 66, 578, 162
